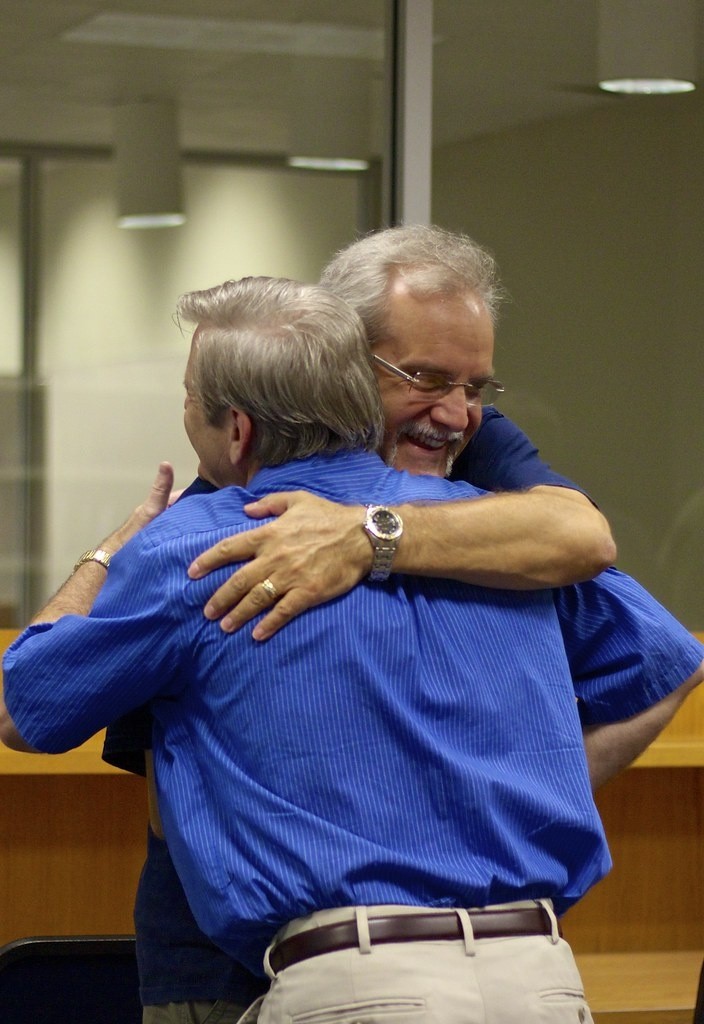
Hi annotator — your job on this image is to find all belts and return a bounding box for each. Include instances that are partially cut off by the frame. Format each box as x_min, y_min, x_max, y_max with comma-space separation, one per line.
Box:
275, 909, 563, 974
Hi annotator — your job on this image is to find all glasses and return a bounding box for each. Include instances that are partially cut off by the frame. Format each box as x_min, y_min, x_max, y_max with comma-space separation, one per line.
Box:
373, 352, 504, 408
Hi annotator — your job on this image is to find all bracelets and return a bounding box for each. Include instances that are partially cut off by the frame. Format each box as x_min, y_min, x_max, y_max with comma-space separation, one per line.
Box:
73, 549, 111, 574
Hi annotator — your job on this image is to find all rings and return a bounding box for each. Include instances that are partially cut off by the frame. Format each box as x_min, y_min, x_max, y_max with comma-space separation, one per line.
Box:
262, 578, 280, 599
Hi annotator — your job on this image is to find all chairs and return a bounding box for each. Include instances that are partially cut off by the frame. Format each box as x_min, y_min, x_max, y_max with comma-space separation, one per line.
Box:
0, 934, 144, 1024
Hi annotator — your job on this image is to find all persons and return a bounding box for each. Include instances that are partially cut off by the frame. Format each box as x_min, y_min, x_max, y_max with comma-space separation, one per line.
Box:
0, 225, 704, 1024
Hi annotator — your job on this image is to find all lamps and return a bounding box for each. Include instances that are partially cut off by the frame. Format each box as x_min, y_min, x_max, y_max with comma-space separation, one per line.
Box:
596, 0, 702, 94
110, 95, 186, 228
288, 21, 372, 170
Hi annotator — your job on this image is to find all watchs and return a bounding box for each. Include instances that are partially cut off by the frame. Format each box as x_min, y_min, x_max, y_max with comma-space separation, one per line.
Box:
364, 503, 404, 581
